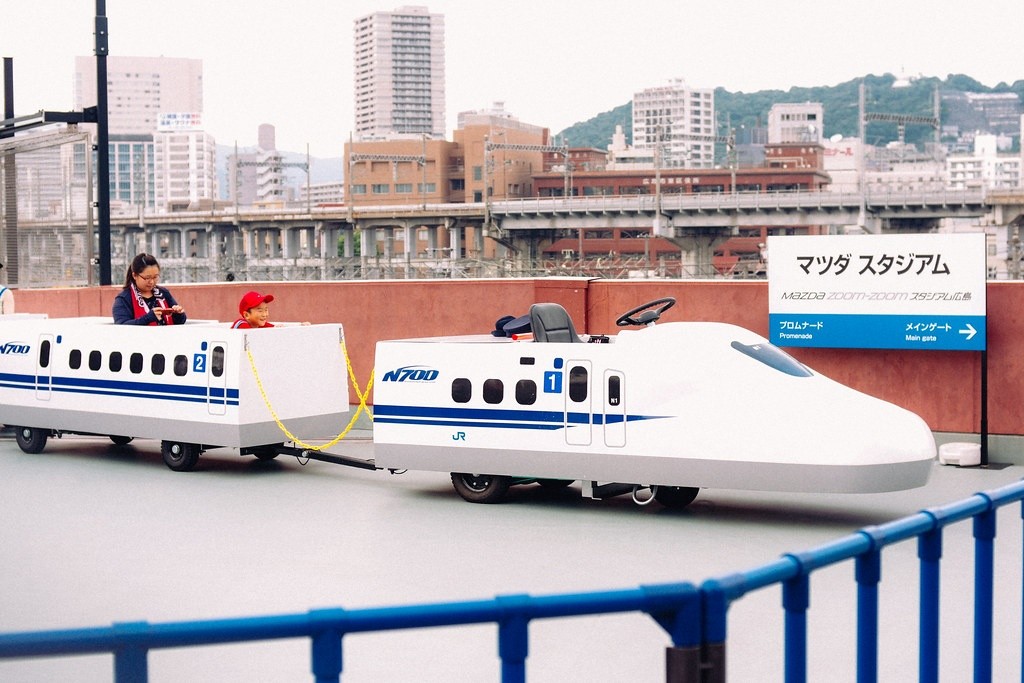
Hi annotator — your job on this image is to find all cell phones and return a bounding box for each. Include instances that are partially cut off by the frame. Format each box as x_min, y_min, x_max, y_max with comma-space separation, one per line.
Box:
157, 308, 174, 315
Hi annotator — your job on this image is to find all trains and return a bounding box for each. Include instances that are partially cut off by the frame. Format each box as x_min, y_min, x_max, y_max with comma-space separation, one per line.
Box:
2, 296, 938, 511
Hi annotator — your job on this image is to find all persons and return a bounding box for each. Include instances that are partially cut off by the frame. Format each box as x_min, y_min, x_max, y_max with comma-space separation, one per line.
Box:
111, 253, 187, 326
231, 291, 277, 329
0, 263, 15, 315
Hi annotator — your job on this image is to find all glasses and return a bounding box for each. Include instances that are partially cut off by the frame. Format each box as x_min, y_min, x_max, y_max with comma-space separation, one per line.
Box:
138, 275, 160, 281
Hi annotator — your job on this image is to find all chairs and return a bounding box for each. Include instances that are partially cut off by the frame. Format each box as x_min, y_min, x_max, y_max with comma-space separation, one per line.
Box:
529, 303, 585, 342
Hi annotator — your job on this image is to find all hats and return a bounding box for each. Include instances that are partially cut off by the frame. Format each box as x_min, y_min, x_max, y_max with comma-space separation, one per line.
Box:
239, 291, 273, 317
503, 314, 530, 337
491, 316, 515, 337
